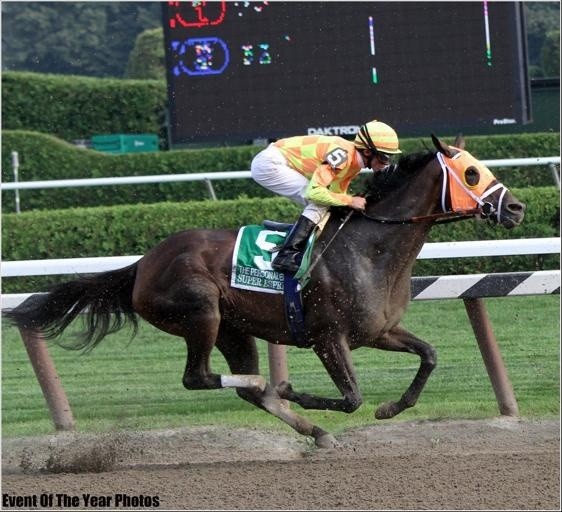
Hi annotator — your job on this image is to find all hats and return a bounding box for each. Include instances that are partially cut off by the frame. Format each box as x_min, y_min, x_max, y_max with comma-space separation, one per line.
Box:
355, 120, 403, 155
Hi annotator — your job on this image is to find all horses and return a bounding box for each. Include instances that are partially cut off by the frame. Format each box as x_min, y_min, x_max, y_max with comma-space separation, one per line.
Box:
1, 130, 527, 450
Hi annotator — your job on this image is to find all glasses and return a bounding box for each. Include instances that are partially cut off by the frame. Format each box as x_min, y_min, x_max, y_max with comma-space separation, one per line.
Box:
374, 151, 393, 163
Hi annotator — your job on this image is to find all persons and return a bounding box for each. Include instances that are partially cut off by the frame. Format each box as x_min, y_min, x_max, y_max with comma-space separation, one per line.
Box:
251, 120, 402, 272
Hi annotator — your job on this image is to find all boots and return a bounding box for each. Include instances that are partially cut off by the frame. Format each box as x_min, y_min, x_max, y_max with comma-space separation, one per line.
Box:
272, 213, 316, 279
